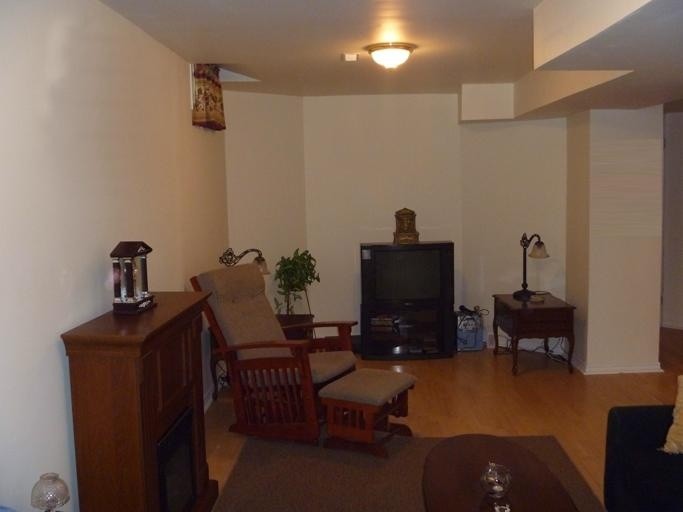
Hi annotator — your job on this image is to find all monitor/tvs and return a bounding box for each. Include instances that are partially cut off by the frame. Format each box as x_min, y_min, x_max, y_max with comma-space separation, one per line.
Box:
360, 241, 455, 306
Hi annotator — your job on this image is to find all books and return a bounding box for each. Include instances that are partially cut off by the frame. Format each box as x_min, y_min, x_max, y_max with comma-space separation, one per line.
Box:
409, 339, 438, 353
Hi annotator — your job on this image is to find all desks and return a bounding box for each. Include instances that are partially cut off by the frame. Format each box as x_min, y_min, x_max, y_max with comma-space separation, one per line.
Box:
489, 293, 578, 375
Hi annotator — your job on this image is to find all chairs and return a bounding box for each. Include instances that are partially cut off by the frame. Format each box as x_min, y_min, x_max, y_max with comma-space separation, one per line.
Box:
191, 263, 358, 446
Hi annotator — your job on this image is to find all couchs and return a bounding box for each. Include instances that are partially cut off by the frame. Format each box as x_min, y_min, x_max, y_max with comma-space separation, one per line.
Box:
597, 404, 682, 509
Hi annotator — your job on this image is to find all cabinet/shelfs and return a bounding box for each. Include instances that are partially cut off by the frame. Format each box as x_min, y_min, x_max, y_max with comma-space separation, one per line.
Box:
61, 292, 218, 511
364, 303, 453, 359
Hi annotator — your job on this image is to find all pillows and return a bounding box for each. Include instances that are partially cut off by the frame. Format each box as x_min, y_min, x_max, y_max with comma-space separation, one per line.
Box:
657, 375, 683, 455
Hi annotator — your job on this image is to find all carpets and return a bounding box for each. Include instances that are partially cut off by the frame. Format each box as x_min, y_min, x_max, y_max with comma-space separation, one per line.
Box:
220, 432, 604, 512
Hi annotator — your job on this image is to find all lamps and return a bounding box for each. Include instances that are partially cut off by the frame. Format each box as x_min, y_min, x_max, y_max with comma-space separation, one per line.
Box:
364, 42, 418, 69
511, 233, 550, 302
220, 246, 270, 275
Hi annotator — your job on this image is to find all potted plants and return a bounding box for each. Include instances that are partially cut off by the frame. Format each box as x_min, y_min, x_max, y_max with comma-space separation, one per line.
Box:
269, 247, 317, 339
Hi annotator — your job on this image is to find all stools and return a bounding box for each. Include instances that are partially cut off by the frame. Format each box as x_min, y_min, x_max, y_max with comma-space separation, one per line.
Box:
315, 363, 415, 457
